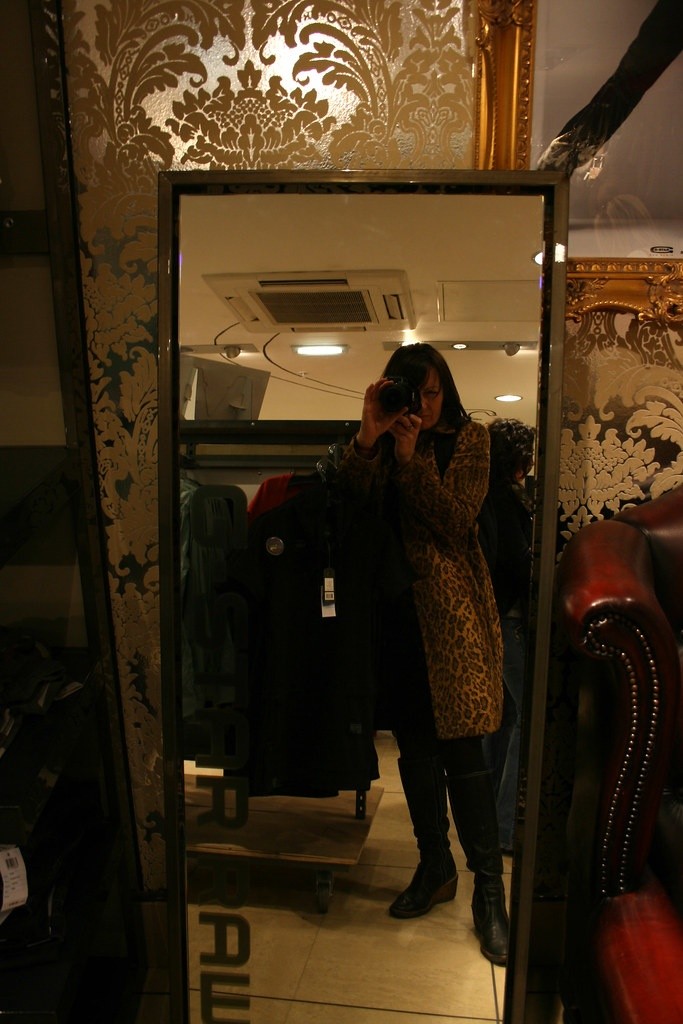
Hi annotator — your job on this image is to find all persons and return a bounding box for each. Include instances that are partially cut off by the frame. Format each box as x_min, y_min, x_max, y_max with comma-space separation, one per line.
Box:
477, 418, 536, 625
336, 342, 510, 965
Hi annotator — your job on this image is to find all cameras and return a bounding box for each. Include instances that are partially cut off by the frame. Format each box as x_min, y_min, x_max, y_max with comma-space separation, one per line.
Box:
379, 380, 423, 418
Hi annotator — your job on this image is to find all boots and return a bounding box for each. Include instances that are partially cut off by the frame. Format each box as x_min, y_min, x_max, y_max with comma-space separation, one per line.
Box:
389, 756, 459, 919
444, 770, 510, 967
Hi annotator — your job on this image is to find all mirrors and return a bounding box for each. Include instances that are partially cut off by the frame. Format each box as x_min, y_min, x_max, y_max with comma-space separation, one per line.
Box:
147, 166, 571, 1024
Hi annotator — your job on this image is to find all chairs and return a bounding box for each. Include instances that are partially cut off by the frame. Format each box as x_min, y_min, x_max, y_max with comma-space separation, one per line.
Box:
558, 482, 683, 1024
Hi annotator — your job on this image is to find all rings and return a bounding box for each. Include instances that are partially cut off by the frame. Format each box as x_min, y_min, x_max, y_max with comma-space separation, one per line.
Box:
407, 423, 414, 431
